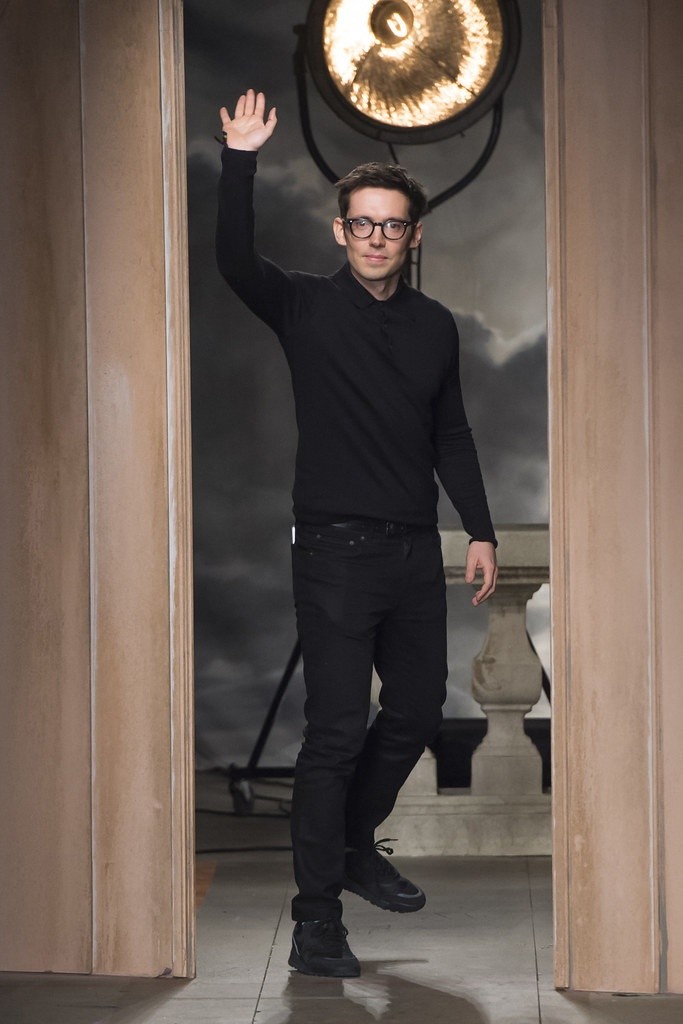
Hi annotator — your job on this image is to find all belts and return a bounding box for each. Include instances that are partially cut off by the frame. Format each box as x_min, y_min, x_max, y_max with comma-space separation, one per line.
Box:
337, 519, 416, 536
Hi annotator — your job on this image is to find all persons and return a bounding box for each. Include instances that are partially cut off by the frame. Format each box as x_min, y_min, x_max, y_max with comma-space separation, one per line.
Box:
216, 88, 499, 982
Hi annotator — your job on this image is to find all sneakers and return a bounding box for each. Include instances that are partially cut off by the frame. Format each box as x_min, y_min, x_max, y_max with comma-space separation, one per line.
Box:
287, 920, 361, 977
343, 844, 426, 914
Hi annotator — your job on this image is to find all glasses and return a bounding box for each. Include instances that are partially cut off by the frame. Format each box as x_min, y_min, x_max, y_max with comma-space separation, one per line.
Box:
341, 216, 417, 241
